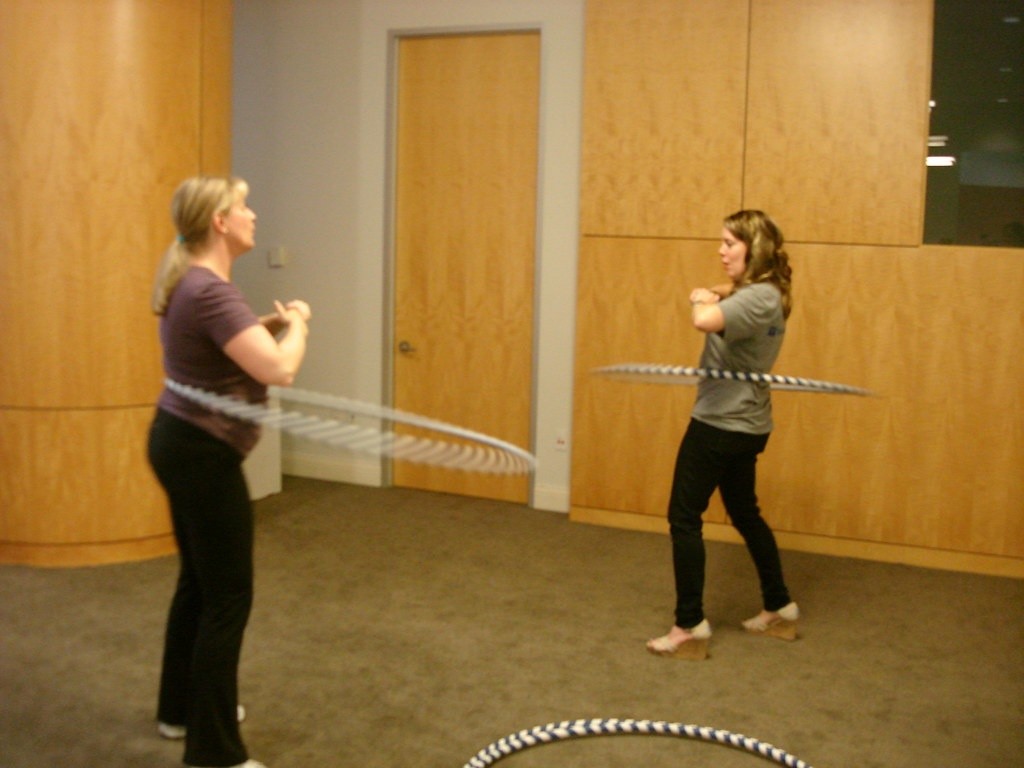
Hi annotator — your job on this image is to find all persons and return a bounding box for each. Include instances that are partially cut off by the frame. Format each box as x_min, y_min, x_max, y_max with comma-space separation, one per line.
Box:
147, 177, 312, 768
648, 210, 799, 660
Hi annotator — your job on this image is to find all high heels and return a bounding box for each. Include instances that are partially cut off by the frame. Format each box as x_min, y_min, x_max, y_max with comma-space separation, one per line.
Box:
741, 602, 799, 640
646, 618, 711, 660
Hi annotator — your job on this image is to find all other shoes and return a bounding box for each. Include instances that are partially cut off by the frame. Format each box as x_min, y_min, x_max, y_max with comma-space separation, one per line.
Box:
231, 758, 267, 768
156, 705, 245, 737
